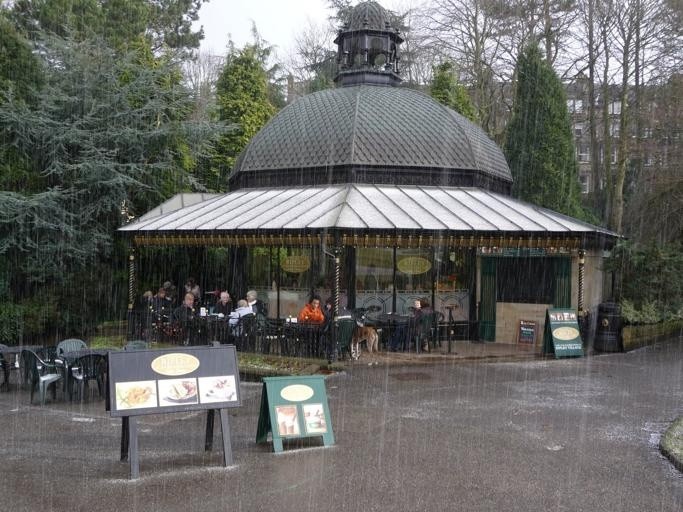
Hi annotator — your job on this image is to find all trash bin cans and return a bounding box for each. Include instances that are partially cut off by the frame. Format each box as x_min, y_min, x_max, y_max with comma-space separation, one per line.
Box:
592, 303, 629, 354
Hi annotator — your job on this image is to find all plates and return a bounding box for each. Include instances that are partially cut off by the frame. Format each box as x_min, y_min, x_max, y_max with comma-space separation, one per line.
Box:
167, 385, 198, 401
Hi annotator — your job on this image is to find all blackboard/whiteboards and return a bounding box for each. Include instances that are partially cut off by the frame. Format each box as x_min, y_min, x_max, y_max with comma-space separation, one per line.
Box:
516, 320, 537, 346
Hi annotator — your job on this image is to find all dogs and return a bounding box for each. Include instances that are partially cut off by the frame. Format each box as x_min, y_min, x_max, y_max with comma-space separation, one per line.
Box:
348, 326, 379, 360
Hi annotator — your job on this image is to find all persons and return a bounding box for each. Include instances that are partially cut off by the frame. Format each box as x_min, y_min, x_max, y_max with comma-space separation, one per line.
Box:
397, 300, 421, 322
299, 294, 324, 354
131, 281, 268, 347
394, 268, 410, 291
388, 296, 432, 353
320, 296, 350, 358
364, 262, 381, 291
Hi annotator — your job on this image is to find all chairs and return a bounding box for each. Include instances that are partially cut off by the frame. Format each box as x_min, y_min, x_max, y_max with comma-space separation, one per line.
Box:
173, 308, 444, 362
0, 339, 148, 407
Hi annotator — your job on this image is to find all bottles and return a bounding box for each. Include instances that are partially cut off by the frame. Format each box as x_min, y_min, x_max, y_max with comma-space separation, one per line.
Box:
289, 312, 292, 319
